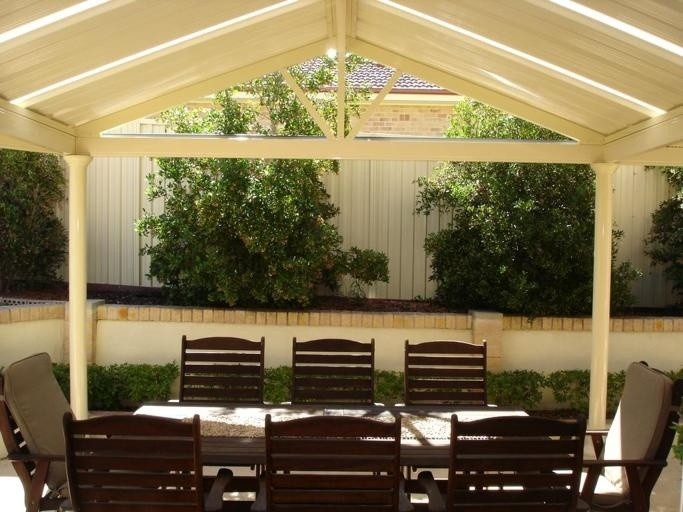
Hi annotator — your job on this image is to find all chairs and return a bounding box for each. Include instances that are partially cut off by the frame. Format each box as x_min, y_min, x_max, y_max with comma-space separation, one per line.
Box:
63, 335, 588, 512
550, 360, 683, 512
1, 352, 72, 511
